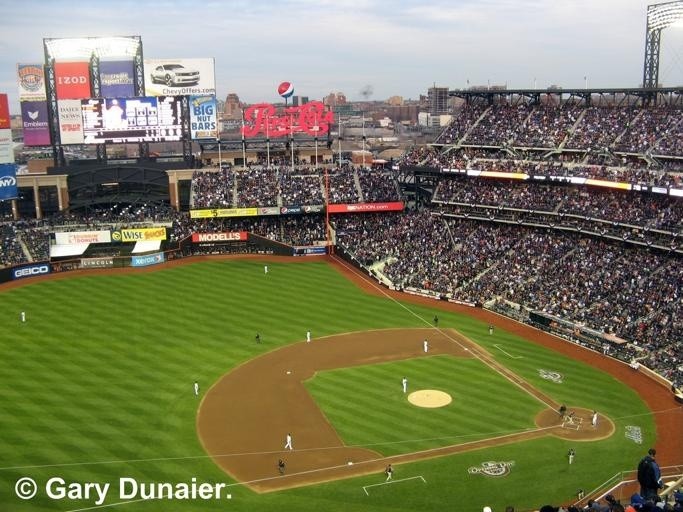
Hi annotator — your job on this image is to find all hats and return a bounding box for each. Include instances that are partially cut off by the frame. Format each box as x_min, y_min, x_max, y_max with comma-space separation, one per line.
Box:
631, 494, 640, 503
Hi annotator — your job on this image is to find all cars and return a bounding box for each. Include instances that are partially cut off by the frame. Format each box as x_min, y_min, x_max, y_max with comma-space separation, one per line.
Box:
150, 64, 200, 87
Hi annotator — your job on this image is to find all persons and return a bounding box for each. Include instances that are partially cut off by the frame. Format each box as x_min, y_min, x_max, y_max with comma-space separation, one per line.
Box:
568, 410, 576, 421
567, 448, 576, 464
591, 410, 598, 426
384, 464, 394, 481
278, 459, 286, 475
637, 448, 664, 496
482, 485, 683, 512
285, 433, 293, 450
558, 403, 566, 419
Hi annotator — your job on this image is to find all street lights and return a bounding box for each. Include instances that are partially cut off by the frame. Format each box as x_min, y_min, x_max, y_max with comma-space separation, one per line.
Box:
643, 0, 683, 89
